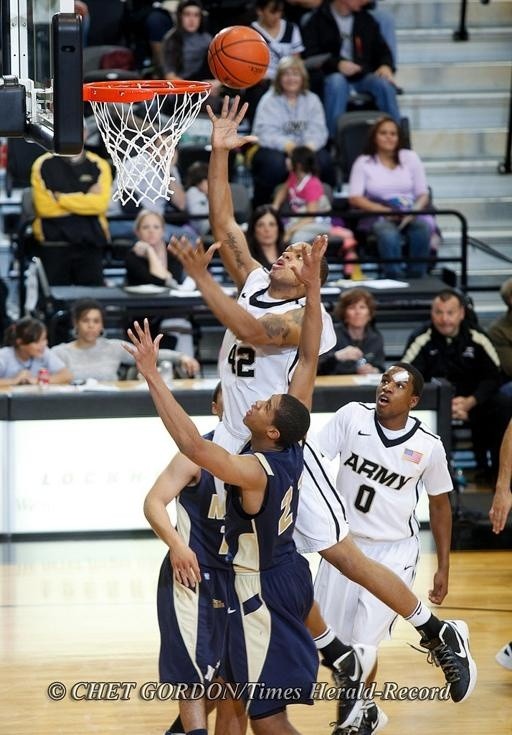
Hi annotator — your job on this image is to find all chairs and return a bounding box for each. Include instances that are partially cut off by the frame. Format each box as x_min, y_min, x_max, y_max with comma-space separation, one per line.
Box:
0, 0, 441, 259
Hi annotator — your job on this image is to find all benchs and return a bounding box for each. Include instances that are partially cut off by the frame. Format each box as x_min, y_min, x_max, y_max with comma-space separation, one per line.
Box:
23, 255, 466, 361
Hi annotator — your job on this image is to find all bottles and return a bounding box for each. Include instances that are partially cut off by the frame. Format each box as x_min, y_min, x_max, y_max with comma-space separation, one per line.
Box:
160, 361, 175, 390
37, 367, 51, 397
356, 357, 367, 370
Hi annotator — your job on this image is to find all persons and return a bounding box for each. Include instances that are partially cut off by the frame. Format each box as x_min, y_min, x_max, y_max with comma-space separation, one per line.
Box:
317, 288, 386, 374
143, 381, 252, 735
0, 318, 72, 386
120, 234, 329, 735
400, 289, 501, 421
310, 364, 455, 693
486, 277, 512, 377
488, 416, 512, 674
50, 300, 201, 382
168, 94, 477, 729
124, 1, 435, 288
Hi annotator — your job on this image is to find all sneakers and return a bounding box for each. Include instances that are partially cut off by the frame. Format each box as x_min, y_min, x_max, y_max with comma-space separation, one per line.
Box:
321, 619, 478, 735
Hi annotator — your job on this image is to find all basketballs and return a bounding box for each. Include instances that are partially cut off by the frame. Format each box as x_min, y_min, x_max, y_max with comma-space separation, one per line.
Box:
209, 25, 270, 89
